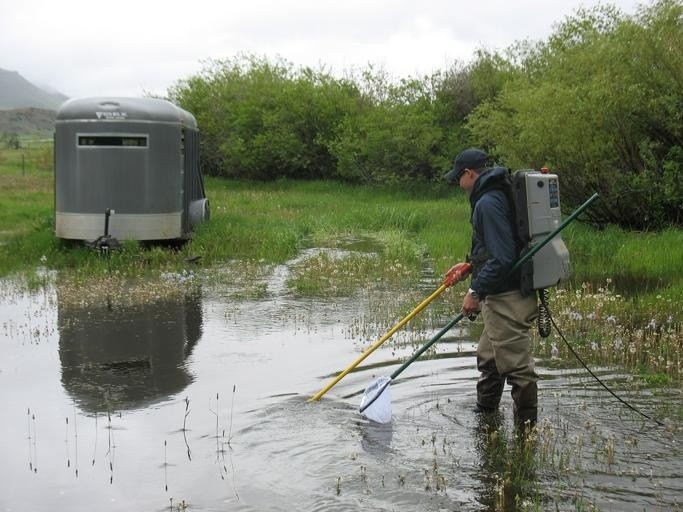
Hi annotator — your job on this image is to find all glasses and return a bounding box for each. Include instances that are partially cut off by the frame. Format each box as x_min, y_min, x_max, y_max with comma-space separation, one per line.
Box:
454, 171, 464, 183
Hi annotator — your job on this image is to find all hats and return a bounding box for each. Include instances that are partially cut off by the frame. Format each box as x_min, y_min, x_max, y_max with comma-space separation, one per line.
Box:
443, 149, 487, 185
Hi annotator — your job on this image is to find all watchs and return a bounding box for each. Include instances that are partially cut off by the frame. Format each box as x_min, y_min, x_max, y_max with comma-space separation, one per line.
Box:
469, 288, 480, 299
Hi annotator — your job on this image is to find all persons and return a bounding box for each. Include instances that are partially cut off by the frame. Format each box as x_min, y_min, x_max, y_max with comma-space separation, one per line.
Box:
443, 149, 543, 426
